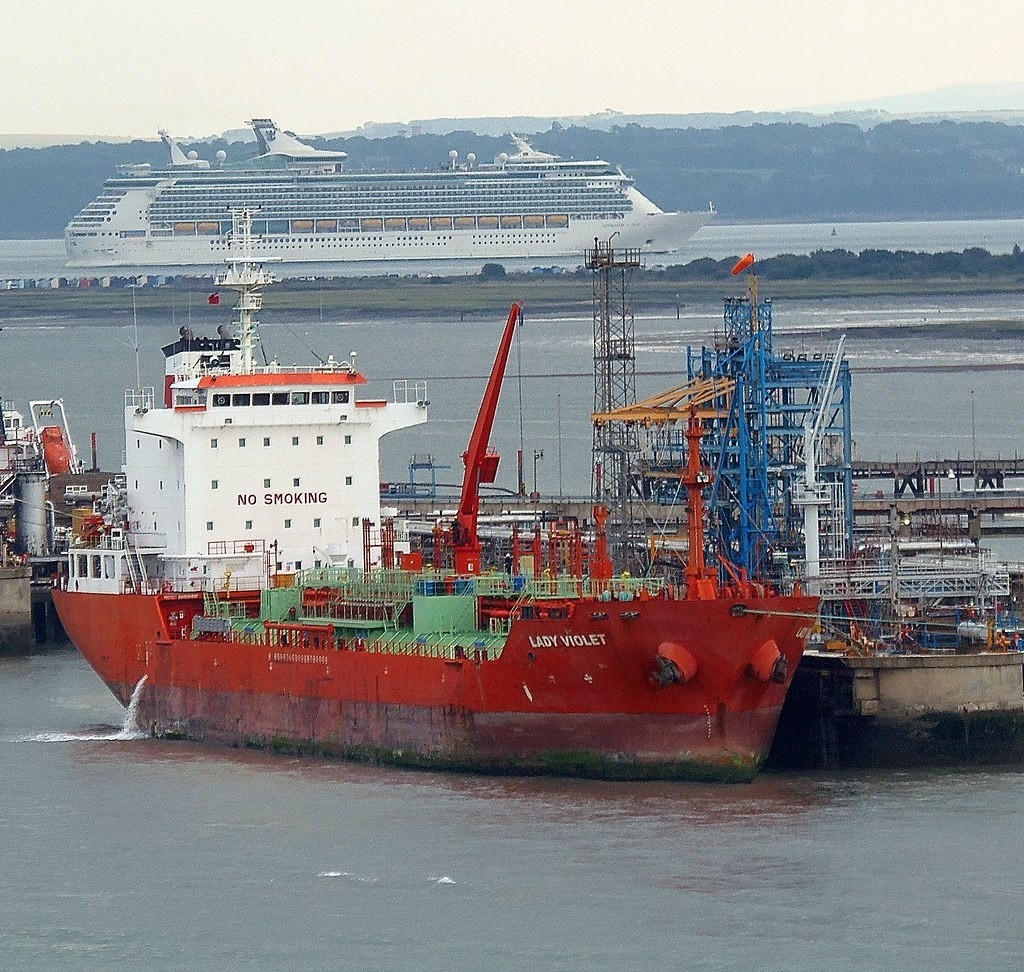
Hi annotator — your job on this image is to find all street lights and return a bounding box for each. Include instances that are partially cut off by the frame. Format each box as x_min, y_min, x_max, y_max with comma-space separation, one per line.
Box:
971, 390, 977, 497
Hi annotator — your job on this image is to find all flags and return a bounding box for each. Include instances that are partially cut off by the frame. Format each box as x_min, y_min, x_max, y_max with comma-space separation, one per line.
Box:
208, 293, 220, 305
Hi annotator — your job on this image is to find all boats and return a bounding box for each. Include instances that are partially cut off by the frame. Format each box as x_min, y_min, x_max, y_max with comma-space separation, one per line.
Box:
547, 216, 567, 223
316, 220, 336, 228
524, 216, 543, 225
199, 222, 218, 232
50, 202, 823, 785
174, 224, 194, 232
409, 218, 429, 226
432, 218, 451, 226
380, 509, 560, 524
454, 218, 474, 226
501, 217, 520, 226
361, 219, 381, 228
402, 520, 597, 543
592, 354, 637, 360
292, 221, 313, 229
478, 217, 498, 225
385, 219, 406, 228
0, 398, 126, 659
789, 648, 1024, 768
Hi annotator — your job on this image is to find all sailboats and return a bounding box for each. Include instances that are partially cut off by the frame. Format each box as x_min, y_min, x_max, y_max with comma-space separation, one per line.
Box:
831, 226, 838, 236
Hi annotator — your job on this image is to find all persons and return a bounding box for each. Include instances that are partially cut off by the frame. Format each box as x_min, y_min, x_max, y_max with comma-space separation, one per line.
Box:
448, 516, 462, 544
505, 553, 512, 574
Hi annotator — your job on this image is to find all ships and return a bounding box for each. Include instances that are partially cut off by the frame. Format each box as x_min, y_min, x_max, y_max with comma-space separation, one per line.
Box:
63, 119, 717, 269
